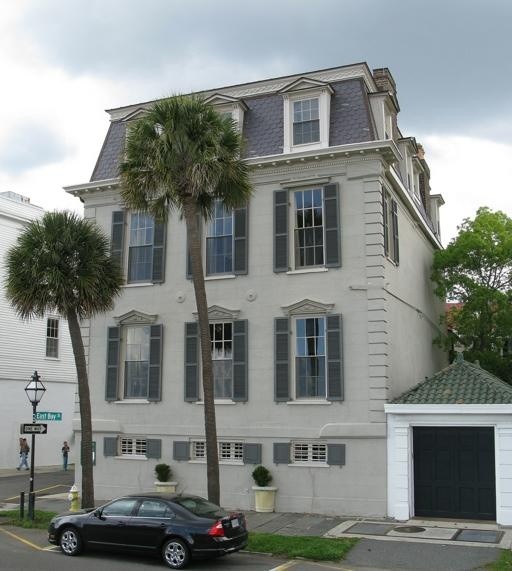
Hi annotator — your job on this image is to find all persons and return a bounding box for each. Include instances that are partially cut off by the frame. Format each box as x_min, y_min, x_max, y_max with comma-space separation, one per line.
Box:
62, 441, 70, 472
16, 438, 30, 470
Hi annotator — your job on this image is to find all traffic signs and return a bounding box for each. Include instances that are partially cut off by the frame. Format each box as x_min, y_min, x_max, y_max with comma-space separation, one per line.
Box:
20, 424, 47, 434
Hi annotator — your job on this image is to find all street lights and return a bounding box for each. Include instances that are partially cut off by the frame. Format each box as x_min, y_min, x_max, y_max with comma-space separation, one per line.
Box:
24, 371, 46, 515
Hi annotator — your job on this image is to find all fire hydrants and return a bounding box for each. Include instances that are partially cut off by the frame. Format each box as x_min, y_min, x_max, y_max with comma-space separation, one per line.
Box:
68, 485, 80, 511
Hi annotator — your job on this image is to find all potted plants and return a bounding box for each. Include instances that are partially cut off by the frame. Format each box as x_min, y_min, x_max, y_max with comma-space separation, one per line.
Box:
251, 466, 278, 513
154, 464, 178, 494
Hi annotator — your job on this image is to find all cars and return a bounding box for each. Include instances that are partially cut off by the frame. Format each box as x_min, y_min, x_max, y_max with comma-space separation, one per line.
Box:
48, 492, 248, 569
